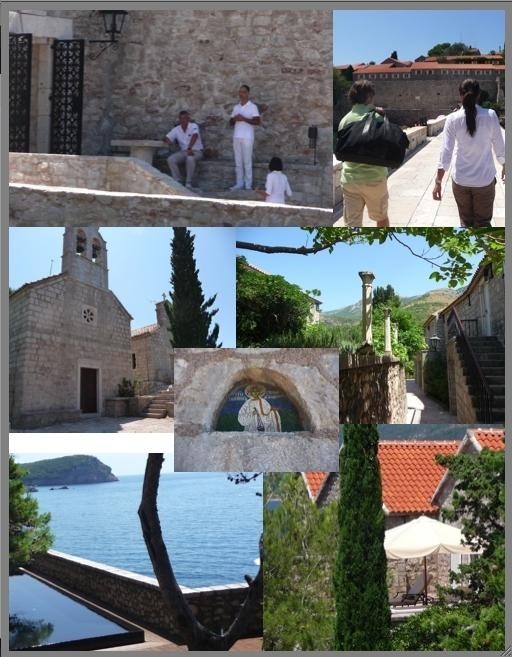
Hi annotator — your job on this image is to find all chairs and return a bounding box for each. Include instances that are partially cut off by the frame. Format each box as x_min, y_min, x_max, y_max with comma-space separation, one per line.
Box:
385, 569, 432, 606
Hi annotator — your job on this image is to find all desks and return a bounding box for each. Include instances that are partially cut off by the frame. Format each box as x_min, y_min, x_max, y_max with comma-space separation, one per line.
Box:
110, 138, 168, 167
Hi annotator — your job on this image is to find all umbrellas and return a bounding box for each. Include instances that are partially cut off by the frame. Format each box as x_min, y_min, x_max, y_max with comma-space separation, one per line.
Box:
384, 513, 483, 605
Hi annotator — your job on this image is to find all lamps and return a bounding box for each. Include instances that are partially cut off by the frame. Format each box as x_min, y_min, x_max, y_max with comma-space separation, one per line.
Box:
430, 334, 442, 351
88, 9, 128, 45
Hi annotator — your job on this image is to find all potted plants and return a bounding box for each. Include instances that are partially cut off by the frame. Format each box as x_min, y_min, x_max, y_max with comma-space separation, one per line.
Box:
105, 375, 152, 416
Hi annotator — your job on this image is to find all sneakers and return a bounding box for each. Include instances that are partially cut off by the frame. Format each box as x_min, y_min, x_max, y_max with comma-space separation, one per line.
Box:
246, 185, 252, 192
229, 184, 244, 191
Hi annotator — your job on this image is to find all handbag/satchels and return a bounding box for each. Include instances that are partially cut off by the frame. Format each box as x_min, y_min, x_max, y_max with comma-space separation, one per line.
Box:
335, 110, 409, 169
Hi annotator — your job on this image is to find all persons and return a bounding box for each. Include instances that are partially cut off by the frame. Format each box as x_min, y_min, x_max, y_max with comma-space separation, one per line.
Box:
255, 157, 292, 204
164, 111, 203, 189
433, 79, 505, 227
229, 85, 260, 191
338, 79, 389, 227
238, 387, 281, 432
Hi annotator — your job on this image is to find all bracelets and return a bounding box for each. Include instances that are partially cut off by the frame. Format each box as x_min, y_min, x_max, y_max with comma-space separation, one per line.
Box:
435, 178, 442, 183
187, 148, 191, 150
